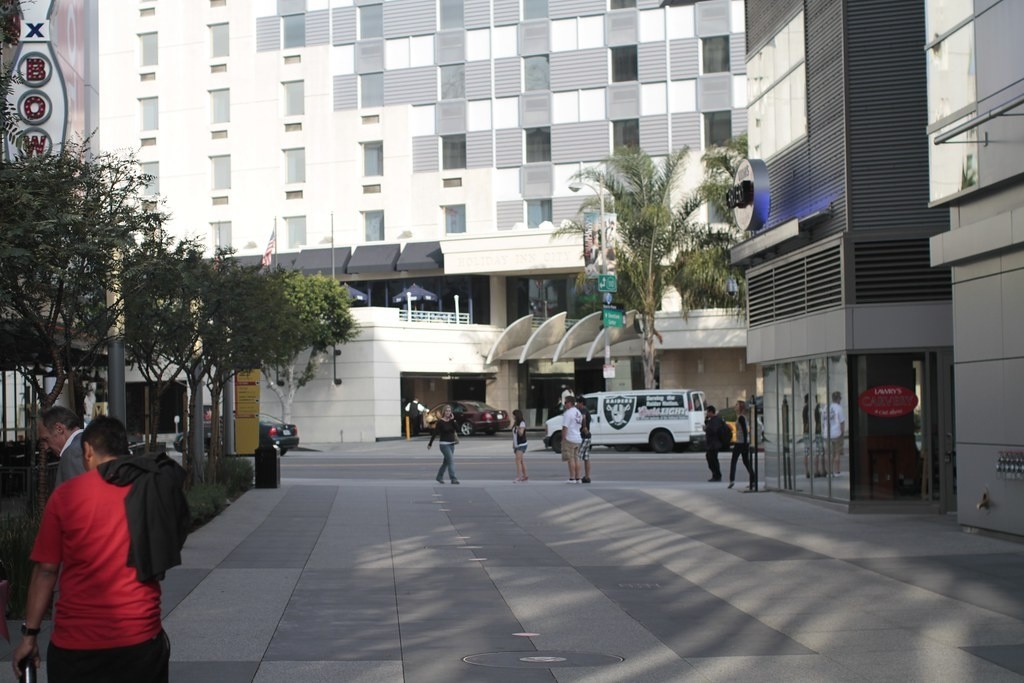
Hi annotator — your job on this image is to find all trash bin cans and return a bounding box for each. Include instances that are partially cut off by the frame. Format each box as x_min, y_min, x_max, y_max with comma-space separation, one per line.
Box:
255, 444, 281, 489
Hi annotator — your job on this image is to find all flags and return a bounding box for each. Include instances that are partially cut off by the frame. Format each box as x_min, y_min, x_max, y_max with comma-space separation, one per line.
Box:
261, 231, 274, 267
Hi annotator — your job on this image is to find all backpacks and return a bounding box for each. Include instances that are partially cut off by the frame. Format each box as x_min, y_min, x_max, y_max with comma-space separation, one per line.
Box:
718, 420, 732, 445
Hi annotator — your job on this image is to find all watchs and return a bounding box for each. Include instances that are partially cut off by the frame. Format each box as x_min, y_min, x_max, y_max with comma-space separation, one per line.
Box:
20, 622, 41, 637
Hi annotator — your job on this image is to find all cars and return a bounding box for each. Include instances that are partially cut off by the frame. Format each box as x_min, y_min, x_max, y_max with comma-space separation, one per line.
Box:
427, 400, 510, 436
173, 411, 301, 456
717, 395, 763, 444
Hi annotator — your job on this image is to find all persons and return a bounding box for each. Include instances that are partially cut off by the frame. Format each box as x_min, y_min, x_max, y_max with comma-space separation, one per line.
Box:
401, 397, 430, 437
37, 406, 87, 636
427, 405, 459, 484
561, 396, 593, 482
705, 405, 724, 481
11, 415, 167, 683
803, 391, 844, 479
727, 399, 757, 489
511, 409, 528, 484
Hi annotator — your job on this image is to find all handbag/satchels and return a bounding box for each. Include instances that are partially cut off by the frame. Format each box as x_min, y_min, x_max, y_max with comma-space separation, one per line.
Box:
454, 430, 459, 444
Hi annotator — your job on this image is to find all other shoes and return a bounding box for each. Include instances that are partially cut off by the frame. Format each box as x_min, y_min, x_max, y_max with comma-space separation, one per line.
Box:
728, 481, 735, 489
436, 478, 444, 483
582, 476, 591, 483
515, 477, 522, 481
575, 478, 582, 484
523, 476, 528, 480
564, 479, 576, 484
708, 477, 721, 482
450, 480, 460, 484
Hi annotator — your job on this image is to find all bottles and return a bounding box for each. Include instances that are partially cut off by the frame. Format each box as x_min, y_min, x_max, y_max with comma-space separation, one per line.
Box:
19, 623, 37, 683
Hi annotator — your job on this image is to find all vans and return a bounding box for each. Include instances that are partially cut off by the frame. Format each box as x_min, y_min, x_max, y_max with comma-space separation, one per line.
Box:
543, 388, 708, 453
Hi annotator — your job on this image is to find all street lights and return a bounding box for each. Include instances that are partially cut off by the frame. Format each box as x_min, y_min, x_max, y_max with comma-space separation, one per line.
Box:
570, 180, 617, 391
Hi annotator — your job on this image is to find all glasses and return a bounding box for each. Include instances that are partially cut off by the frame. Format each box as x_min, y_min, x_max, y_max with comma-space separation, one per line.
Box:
564, 401, 568, 404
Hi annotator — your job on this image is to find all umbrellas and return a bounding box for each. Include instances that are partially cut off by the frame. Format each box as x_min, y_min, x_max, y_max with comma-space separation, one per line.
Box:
344, 284, 367, 306
393, 283, 439, 322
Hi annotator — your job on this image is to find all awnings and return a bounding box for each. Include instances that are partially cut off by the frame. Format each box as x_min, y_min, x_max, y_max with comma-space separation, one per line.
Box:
221, 242, 444, 277
